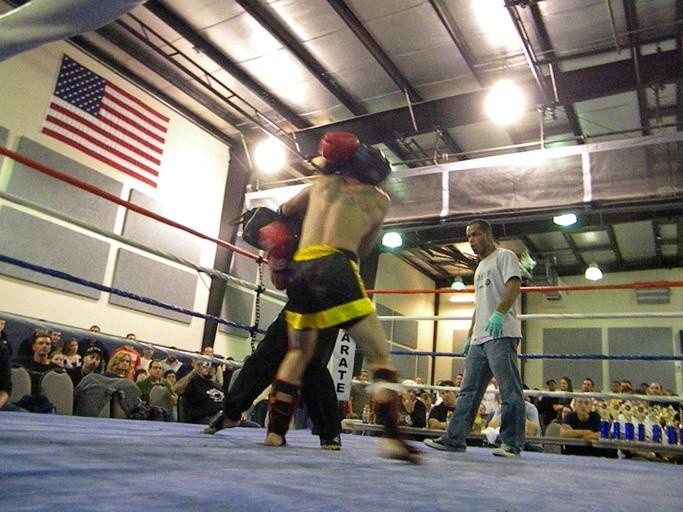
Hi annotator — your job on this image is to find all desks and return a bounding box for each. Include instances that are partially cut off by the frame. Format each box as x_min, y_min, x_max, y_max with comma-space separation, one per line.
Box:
341, 415, 682, 465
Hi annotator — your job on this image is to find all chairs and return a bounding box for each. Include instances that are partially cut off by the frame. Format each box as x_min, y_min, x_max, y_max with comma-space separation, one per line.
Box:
6, 363, 186, 424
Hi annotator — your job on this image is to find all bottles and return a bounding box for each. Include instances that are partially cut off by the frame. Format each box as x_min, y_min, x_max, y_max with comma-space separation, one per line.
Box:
599, 420, 682, 444
445, 410, 454, 430
361, 403, 371, 424
474, 415, 482, 432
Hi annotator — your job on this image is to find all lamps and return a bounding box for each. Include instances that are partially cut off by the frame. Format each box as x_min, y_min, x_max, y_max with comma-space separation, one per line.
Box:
584, 261, 603, 281
451, 264, 465, 290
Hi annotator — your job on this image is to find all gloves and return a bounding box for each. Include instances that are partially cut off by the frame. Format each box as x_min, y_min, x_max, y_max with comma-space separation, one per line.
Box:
321, 132, 360, 164
462, 337, 470, 355
485, 311, 504, 340
258, 221, 294, 258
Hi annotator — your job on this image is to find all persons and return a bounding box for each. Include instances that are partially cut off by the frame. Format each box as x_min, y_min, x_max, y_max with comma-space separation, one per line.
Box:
262, 147, 425, 466
422, 217, 525, 457
2, 318, 315, 430
336, 366, 683, 464
199, 130, 361, 449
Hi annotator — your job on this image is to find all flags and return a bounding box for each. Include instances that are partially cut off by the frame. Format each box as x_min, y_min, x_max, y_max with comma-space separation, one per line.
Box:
41, 52, 168, 190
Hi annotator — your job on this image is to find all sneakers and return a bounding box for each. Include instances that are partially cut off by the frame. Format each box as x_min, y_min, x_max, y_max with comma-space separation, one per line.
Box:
424, 437, 466, 451
492, 444, 520, 457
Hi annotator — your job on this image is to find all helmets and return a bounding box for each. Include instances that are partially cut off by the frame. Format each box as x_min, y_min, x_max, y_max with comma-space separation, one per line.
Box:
241, 207, 283, 251
346, 142, 391, 184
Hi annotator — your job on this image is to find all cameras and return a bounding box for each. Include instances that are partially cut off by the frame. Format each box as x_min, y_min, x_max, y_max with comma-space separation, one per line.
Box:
202, 360, 211, 368
